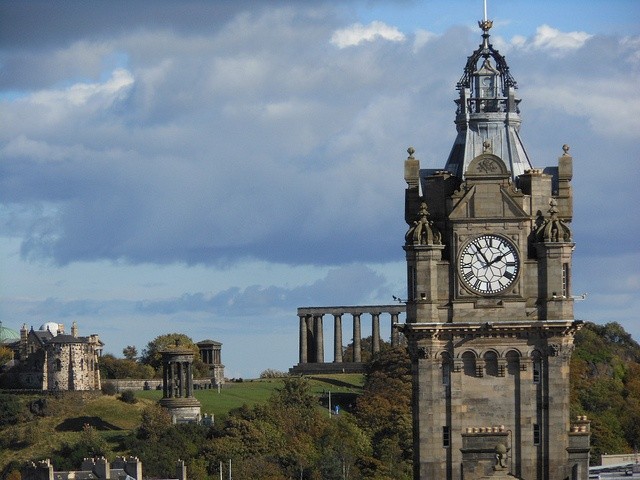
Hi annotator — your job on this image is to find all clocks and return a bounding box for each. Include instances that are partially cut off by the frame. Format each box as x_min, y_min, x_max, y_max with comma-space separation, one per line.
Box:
456, 233, 523, 297
